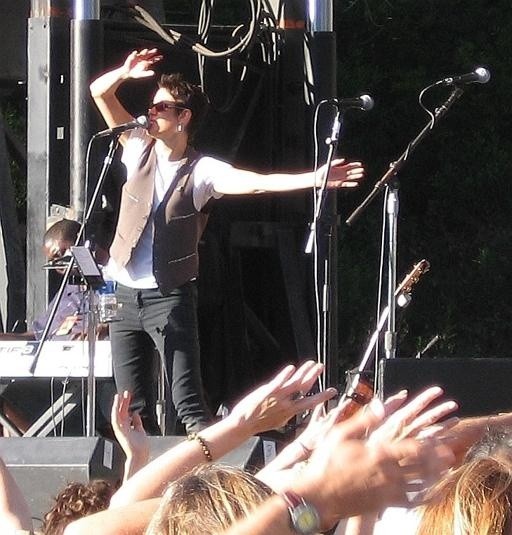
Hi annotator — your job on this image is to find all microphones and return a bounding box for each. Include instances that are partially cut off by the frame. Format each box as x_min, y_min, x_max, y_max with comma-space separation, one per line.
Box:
327, 95, 375, 112
439, 67, 490, 85
95, 114, 149, 139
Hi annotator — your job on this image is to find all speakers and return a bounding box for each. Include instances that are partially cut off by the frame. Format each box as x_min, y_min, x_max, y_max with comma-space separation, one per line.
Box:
145, 435, 265, 487
1, 436, 124, 531
380, 356, 512, 418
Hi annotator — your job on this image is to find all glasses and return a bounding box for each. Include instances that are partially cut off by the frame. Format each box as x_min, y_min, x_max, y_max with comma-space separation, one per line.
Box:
149, 102, 185, 111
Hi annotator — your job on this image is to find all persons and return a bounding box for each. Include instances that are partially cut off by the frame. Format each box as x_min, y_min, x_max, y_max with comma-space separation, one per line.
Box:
1, 217, 118, 437
0, 352, 512, 535
86, 46, 366, 434
